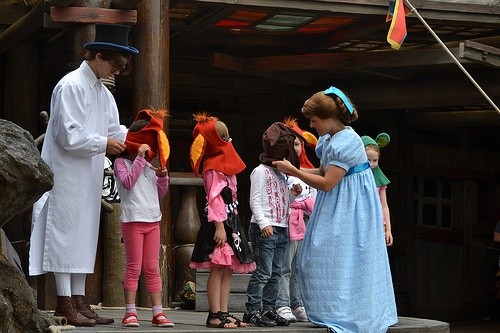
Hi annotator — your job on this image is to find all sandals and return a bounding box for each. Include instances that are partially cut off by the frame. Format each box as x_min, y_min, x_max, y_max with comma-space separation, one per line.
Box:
206, 309, 248, 328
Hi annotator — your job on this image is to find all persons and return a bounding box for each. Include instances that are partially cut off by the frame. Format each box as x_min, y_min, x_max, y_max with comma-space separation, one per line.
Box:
189, 111, 258, 327
275, 119, 318, 324
299, 85, 399, 332
358, 132, 395, 247
28, 23, 141, 328
113, 108, 176, 327
242, 121, 303, 329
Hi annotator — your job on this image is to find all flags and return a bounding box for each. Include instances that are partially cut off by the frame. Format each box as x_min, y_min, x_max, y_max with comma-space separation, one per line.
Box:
385, 0, 414, 50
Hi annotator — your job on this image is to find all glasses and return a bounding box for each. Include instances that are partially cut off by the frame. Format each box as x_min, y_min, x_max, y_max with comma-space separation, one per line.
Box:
102, 55, 127, 73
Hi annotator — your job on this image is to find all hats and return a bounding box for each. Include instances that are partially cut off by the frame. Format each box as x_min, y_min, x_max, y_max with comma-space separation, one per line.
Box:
84, 23, 140, 55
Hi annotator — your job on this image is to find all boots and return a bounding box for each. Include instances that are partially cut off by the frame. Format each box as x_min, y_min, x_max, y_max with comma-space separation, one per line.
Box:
54, 294, 115, 327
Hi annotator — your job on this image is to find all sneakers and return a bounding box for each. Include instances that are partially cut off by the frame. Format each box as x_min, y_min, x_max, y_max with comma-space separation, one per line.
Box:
263, 308, 290, 326
244, 311, 277, 327
291, 305, 310, 322
276, 306, 296, 322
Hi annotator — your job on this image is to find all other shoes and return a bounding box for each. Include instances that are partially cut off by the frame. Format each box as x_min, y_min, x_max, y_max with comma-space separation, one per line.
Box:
151, 313, 175, 326
122, 312, 140, 327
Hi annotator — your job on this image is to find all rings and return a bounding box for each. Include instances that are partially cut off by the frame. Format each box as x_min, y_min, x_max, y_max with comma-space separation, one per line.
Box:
112, 147, 116, 150
